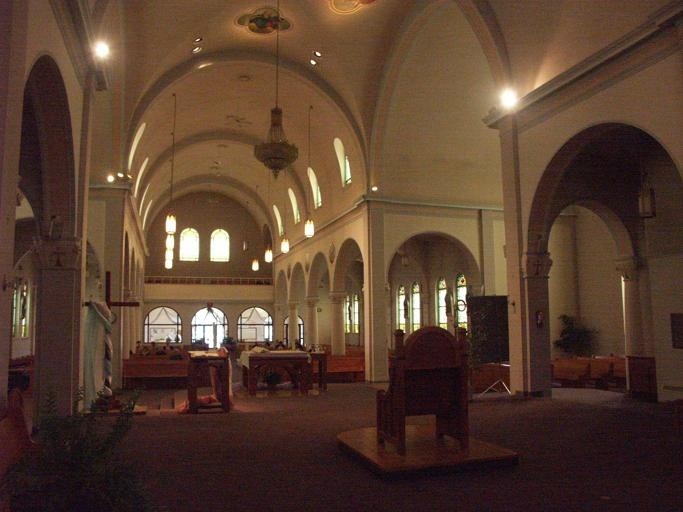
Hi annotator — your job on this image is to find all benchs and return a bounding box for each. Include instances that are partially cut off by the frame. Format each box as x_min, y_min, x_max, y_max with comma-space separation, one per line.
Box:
551, 352, 625, 388
122, 343, 193, 385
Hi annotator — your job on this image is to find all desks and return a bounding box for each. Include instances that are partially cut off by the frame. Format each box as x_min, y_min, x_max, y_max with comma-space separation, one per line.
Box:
240, 350, 327, 397
187, 346, 232, 416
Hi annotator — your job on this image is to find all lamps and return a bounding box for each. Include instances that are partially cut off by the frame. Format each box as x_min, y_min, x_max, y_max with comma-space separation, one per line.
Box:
634, 133, 657, 219
252, 1, 301, 180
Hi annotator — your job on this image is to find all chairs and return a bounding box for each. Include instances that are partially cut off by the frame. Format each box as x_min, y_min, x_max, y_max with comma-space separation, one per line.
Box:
373, 325, 474, 457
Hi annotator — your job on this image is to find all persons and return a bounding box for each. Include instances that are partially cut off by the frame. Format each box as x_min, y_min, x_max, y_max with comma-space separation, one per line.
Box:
252, 341, 303, 351
136, 340, 180, 356
